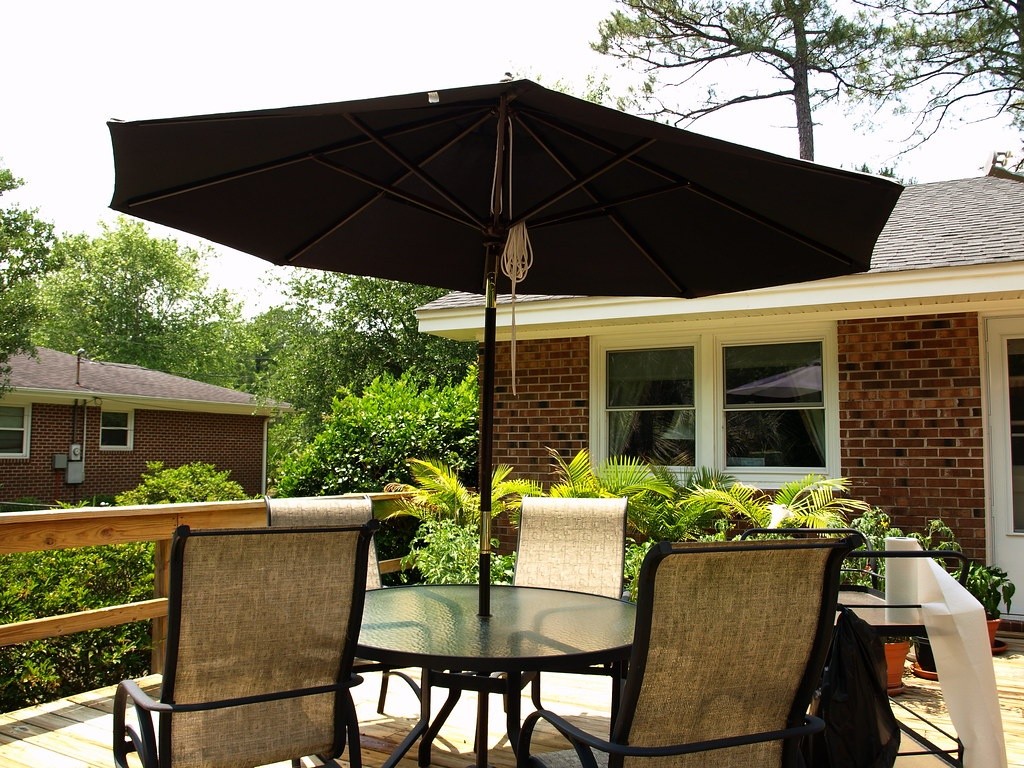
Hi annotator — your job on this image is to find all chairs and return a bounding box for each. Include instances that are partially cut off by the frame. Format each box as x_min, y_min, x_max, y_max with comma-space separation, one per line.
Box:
517, 533, 863, 768
503, 495, 630, 714
113, 518, 381, 768
263, 495, 431, 716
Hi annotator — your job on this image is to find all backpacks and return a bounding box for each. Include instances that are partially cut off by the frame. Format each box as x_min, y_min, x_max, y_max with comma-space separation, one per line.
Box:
799, 609, 902, 768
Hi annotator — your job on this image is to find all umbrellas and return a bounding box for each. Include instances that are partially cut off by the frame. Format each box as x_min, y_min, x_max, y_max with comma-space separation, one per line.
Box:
107, 72, 906, 768
727, 358, 823, 397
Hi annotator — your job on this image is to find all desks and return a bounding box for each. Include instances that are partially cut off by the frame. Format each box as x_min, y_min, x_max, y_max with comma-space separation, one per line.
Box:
354, 585, 638, 767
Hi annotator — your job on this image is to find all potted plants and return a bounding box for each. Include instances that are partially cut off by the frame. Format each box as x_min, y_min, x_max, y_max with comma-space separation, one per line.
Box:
838, 506, 1016, 696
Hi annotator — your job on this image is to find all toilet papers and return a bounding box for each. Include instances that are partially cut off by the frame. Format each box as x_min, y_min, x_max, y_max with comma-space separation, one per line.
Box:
882, 536, 1008, 768
